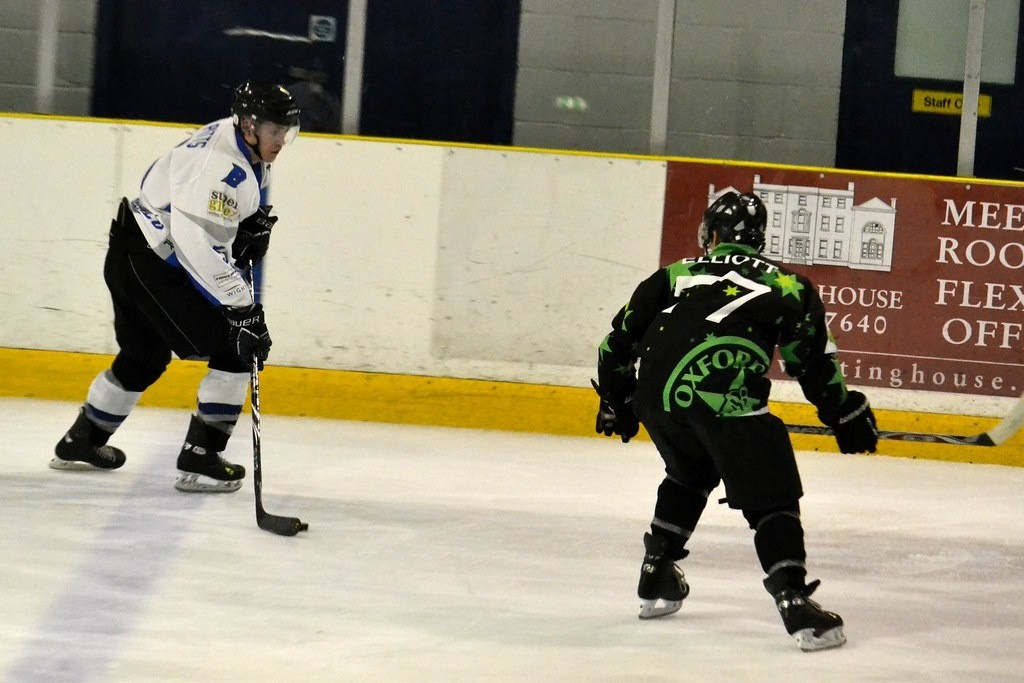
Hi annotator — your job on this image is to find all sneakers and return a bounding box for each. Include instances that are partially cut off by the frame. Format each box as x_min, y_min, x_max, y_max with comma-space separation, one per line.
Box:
637, 532, 689, 619
763, 566, 845, 652
49, 406, 126, 472
174, 415, 245, 493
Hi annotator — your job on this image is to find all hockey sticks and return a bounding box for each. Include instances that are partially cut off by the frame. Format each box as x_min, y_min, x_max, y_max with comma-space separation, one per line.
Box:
598, 390, 1024, 447
247, 258, 302, 538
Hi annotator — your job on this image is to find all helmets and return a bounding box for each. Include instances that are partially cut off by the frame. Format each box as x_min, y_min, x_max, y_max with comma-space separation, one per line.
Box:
231, 77, 299, 135
705, 192, 767, 246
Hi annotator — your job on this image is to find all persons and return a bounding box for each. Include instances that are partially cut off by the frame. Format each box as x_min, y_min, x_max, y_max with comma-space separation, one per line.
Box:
590, 194, 879, 652
51, 69, 299, 494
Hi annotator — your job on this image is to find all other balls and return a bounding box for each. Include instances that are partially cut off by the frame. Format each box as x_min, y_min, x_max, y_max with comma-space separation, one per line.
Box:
299, 523, 309, 532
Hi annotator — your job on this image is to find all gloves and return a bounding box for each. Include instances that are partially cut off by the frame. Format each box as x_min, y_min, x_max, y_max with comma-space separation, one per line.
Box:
591, 378, 639, 443
222, 303, 272, 371
818, 390, 878, 455
231, 206, 278, 267
